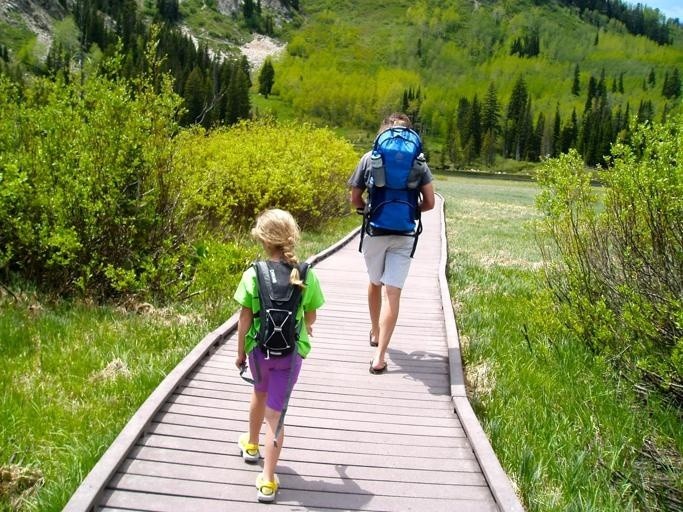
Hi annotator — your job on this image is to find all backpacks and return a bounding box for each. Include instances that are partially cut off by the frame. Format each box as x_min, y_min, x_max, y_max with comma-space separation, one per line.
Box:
357, 126, 426, 237
251, 261, 309, 360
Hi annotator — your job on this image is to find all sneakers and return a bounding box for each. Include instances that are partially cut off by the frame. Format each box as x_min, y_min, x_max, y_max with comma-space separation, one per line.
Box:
256, 473, 279, 501
239, 433, 259, 462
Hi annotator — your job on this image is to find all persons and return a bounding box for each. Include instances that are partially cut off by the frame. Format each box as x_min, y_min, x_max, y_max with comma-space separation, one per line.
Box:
233, 209, 324, 501
349, 111, 435, 375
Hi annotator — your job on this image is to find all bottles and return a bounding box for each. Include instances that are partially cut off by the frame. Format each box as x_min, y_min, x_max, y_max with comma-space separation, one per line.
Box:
369, 151, 386, 189
405, 153, 424, 191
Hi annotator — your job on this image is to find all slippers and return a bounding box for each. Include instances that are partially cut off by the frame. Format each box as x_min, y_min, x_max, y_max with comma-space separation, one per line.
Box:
370, 329, 378, 347
369, 358, 387, 375
